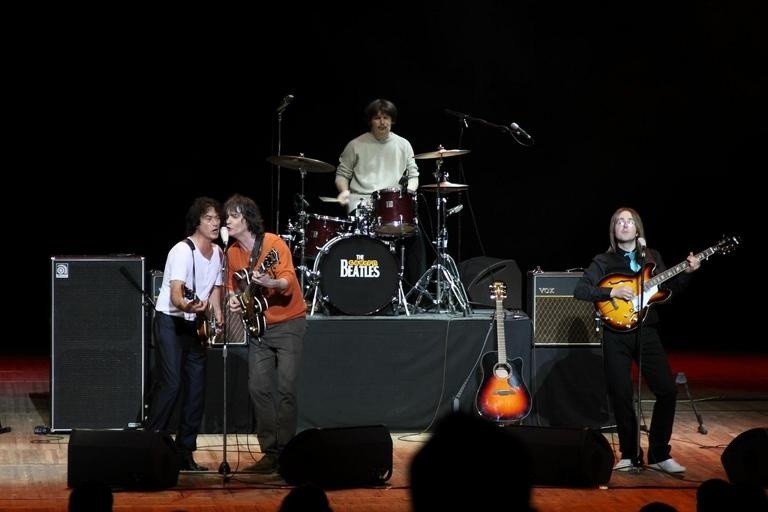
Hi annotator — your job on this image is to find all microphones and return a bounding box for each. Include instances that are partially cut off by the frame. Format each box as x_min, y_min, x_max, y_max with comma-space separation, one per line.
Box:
399, 169, 410, 191
511, 121, 533, 141
638, 238, 648, 268
221, 226, 230, 245
284, 94, 294, 108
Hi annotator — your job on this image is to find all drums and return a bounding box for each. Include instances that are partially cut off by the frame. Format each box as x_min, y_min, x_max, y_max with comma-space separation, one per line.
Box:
371, 187, 418, 240
294, 213, 346, 261
311, 233, 403, 316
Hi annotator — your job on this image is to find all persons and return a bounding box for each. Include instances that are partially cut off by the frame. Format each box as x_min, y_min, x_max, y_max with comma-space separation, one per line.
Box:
335, 99, 421, 315
573, 207, 700, 475
147, 196, 225, 470
222, 195, 307, 477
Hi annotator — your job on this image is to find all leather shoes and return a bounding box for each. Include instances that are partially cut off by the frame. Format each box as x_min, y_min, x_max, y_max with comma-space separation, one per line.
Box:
242, 455, 280, 477
179, 451, 208, 471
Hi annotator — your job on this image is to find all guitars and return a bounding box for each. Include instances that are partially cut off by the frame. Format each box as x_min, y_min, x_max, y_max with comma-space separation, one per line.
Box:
237, 248, 279, 338
592, 237, 738, 333
182, 284, 217, 352
473, 282, 532, 423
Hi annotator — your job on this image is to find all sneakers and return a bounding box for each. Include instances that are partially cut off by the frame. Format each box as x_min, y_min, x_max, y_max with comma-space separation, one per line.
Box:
648, 459, 687, 474
612, 459, 643, 471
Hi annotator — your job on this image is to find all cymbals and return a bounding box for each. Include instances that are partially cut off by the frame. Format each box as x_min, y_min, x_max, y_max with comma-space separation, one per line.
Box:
412, 149, 470, 159
268, 156, 335, 173
420, 181, 470, 194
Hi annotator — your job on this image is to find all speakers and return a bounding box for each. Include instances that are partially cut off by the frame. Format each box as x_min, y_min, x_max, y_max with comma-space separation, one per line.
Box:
67, 429, 180, 494
504, 427, 616, 492
51, 254, 146, 431
531, 269, 608, 416
280, 425, 396, 489
